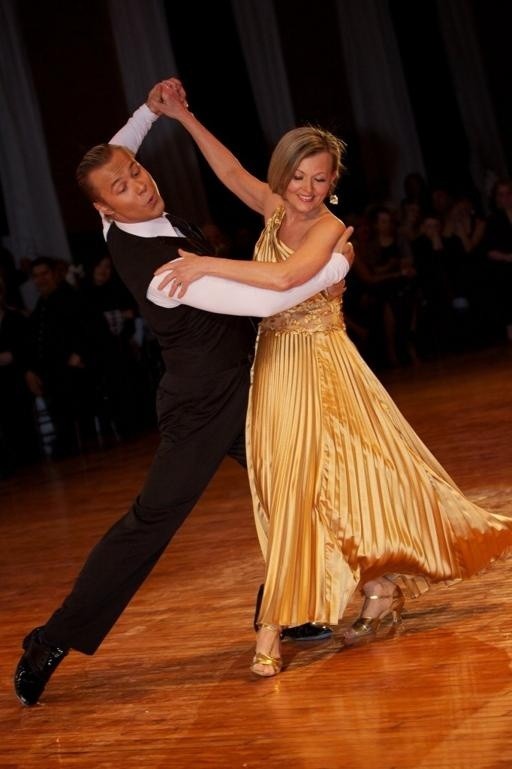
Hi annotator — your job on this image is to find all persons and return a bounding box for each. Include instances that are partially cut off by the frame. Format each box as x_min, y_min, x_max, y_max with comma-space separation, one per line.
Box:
146, 79, 510, 679
13, 79, 357, 705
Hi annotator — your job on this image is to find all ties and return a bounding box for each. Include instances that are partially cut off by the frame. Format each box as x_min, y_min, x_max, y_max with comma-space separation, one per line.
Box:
165, 215, 214, 256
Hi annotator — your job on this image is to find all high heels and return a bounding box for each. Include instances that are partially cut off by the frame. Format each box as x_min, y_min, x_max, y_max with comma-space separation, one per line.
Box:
249, 624, 283, 677
343, 585, 405, 638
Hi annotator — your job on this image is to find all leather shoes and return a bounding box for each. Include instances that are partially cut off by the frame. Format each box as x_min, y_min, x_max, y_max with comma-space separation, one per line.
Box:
14, 627, 68, 706
254, 611, 333, 641
